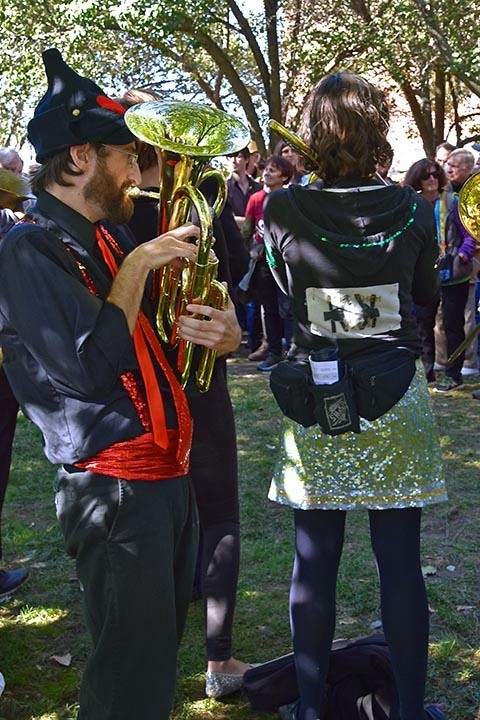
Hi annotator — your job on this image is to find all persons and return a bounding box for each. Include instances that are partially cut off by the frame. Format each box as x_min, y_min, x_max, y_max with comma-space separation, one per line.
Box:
0, 148, 29, 505
433, 141, 455, 164
115, 87, 261, 700
225, 149, 263, 355
377, 138, 400, 184
403, 158, 476, 394
254, 72, 449, 717
242, 154, 297, 371
249, 138, 307, 360
431, 149, 479, 376
2, 51, 242, 720
245, 137, 265, 180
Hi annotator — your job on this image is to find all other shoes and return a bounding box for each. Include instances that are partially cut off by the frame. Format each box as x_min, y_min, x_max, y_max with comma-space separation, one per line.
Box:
205, 663, 265, 699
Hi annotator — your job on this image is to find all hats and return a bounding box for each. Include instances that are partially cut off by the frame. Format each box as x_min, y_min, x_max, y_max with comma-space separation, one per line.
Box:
25, 47, 137, 166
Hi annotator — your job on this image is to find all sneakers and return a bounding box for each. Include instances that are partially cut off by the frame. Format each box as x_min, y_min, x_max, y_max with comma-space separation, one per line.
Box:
432, 362, 446, 371
432, 373, 467, 393
0, 566, 31, 598
459, 367, 480, 376
257, 353, 285, 371
247, 339, 274, 361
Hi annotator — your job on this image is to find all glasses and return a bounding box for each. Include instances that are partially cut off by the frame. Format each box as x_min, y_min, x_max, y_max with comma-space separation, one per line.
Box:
421, 169, 440, 181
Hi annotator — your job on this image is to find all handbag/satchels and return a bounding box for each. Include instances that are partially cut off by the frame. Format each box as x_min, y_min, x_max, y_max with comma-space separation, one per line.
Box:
264, 343, 418, 437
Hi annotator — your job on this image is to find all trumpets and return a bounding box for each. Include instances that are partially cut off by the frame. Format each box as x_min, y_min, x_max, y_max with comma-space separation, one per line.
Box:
268, 118, 327, 176
450, 168, 480, 243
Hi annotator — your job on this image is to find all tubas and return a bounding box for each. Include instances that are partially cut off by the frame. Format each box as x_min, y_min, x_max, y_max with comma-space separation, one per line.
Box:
124, 100, 251, 396
0, 167, 36, 209
